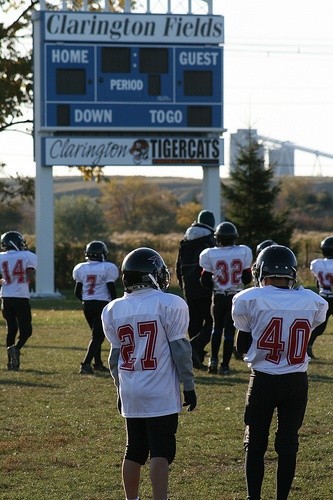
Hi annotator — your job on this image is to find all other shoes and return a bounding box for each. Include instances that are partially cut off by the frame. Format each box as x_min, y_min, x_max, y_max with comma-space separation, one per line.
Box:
6, 345, 22, 370
207, 359, 219, 375
307, 344, 320, 360
219, 362, 230, 375
232, 346, 244, 361
192, 351, 207, 370
80, 361, 95, 375
93, 363, 110, 372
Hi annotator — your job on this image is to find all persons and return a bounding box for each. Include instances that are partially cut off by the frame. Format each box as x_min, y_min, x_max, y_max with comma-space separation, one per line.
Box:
306, 236, 333, 359
0, 231, 38, 371
176, 210, 221, 371
100, 246, 197, 500
232, 244, 328, 500
199, 222, 254, 375
233, 240, 278, 361
73, 240, 119, 375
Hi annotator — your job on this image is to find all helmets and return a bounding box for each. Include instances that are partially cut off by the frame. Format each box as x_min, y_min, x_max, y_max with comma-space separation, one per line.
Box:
120, 247, 170, 292
214, 221, 239, 241
255, 240, 278, 257
0, 231, 30, 252
198, 210, 215, 227
320, 236, 333, 259
251, 245, 300, 283
84, 240, 109, 262
